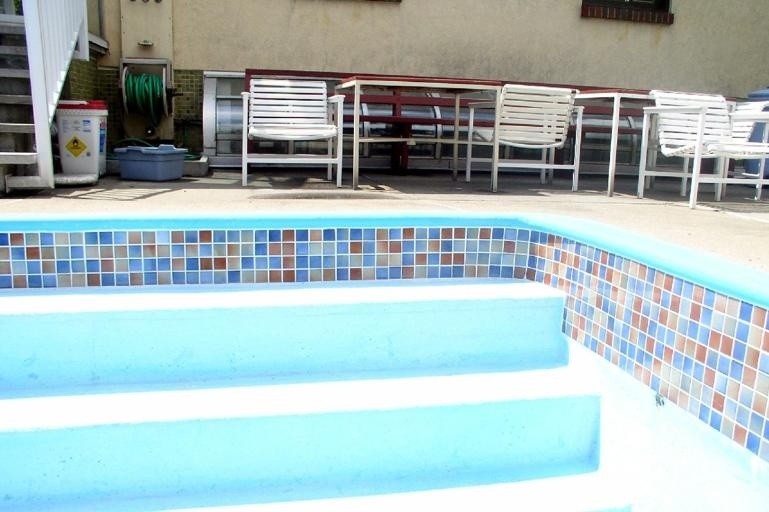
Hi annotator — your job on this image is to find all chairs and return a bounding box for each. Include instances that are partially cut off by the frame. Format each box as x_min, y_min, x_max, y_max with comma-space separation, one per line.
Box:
240, 78, 347, 190
634, 89, 769, 209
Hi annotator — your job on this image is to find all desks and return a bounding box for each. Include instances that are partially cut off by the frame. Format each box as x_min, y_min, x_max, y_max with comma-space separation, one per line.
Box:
336, 77, 653, 197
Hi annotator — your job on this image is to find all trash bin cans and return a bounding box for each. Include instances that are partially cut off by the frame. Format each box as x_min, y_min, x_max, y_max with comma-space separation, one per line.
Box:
56, 100, 109, 177
744, 86, 769, 186
